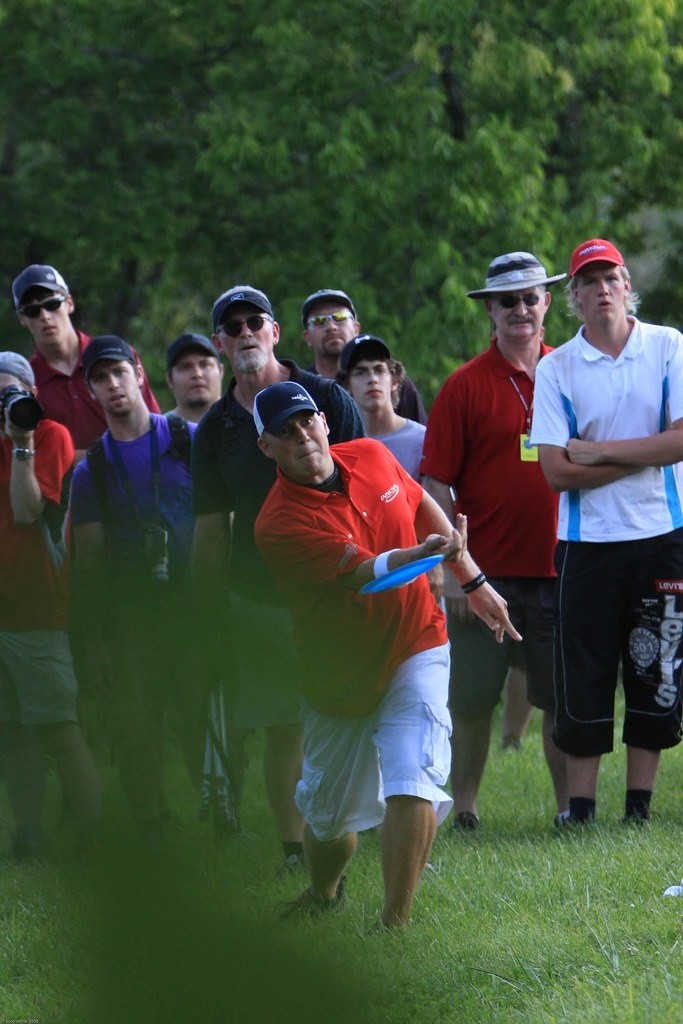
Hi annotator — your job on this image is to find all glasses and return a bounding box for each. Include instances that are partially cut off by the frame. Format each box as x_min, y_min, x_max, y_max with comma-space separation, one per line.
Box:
216, 315, 273, 337
488, 293, 545, 308
18, 297, 67, 318
306, 312, 355, 329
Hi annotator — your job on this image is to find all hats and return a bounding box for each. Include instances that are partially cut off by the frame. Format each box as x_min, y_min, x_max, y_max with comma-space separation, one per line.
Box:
12, 265, 69, 308
253, 381, 320, 436
167, 334, 218, 370
340, 334, 391, 373
82, 335, 135, 383
302, 290, 355, 330
212, 286, 274, 334
570, 239, 624, 278
0, 351, 35, 387
466, 252, 566, 299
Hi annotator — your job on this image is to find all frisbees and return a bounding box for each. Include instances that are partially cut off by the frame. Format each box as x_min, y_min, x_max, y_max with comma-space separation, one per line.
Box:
359, 553, 445, 593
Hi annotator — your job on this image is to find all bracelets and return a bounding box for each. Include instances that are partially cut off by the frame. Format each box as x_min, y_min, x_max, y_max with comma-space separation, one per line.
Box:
460, 572, 487, 594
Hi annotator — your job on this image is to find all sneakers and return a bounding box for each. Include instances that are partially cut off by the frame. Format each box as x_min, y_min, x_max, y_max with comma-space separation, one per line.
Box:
274, 874, 342, 927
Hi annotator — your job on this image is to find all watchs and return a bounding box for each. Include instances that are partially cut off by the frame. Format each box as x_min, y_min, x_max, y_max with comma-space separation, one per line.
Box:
12, 448, 35, 460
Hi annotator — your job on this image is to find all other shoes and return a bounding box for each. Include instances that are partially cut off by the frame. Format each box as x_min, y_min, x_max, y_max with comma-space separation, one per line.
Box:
553, 811, 574, 827
453, 812, 478, 829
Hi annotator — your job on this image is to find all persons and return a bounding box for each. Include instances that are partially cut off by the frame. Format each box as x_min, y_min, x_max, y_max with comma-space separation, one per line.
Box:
0, 252, 570, 833
528, 239, 683, 828
254, 380, 523, 934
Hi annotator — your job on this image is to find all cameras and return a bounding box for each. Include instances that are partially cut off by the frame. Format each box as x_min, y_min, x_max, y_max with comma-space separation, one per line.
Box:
0, 384, 43, 431
